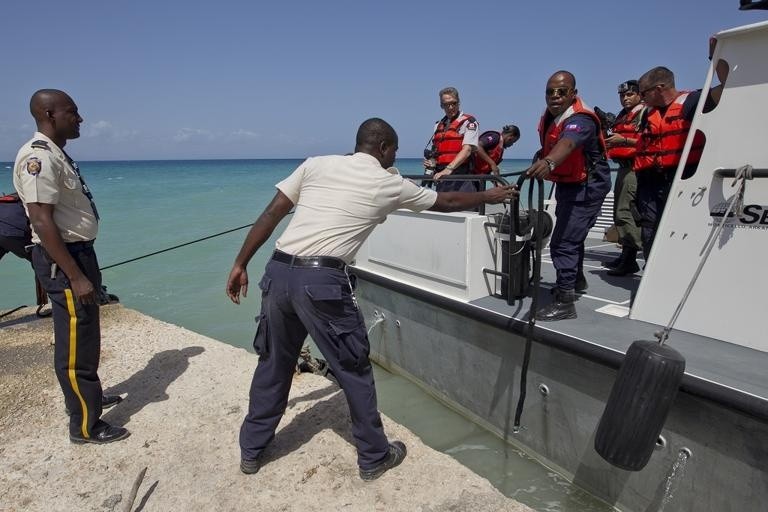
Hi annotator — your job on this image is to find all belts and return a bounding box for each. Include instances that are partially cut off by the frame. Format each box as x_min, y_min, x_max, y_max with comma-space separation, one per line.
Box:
270, 249, 347, 270
65, 240, 94, 251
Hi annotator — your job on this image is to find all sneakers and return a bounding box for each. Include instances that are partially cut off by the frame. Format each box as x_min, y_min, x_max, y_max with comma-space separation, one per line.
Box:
241, 456, 262, 473
359, 441, 407, 481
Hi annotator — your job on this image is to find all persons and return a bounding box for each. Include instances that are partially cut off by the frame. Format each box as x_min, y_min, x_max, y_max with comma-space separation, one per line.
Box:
423, 85, 481, 213
0, 190, 35, 273
474, 125, 520, 214
224, 118, 521, 482
603, 78, 647, 277
633, 35, 729, 271
11, 87, 131, 445
524, 69, 612, 323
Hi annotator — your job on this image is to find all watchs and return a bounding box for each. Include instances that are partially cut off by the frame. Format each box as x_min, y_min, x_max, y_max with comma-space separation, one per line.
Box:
542, 157, 557, 173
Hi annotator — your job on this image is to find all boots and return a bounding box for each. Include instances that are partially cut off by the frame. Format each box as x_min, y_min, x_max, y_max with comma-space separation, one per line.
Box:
551, 267, 588, 294
605, 254, 621, 268
535, 289, 577, 321
607, 246, 640, 276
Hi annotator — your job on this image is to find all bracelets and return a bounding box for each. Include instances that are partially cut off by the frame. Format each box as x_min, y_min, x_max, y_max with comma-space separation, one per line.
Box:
708, 56, 712, 61
623, 137, 628, 146
444, 165, 454, 170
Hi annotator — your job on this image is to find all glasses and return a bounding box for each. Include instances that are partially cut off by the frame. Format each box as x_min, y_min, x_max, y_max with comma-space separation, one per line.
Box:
441, 101, 458, 108
546, 87, 569, 96
638, 83, 665, 97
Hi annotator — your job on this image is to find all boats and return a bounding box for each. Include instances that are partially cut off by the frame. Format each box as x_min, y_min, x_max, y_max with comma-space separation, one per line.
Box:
348, 3, 768, 511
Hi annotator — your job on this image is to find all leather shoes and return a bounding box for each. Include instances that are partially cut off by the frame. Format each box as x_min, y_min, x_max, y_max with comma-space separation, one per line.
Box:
65, 395, 122, 415
70, 426, 129, 445
